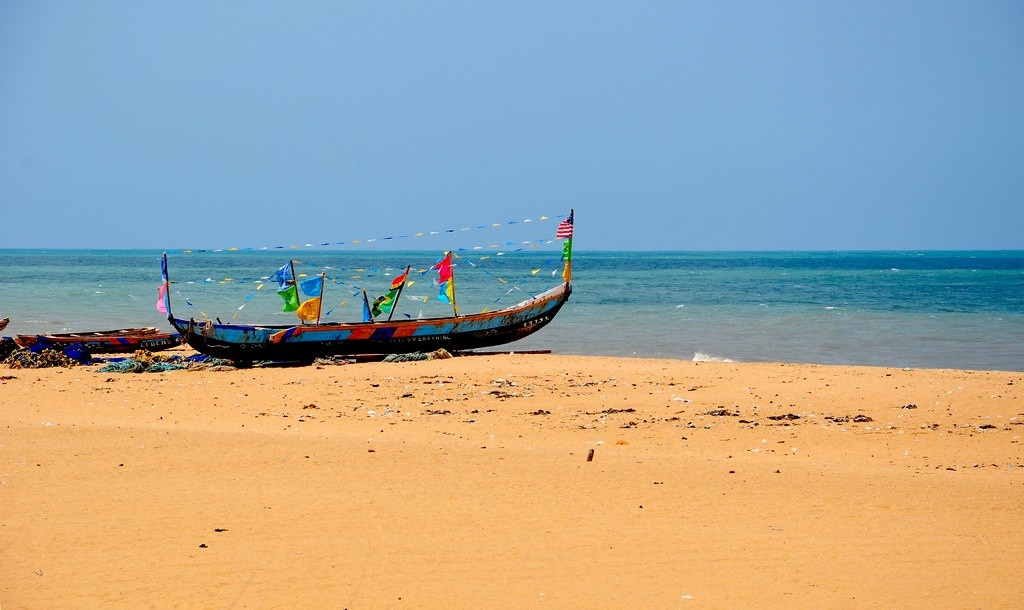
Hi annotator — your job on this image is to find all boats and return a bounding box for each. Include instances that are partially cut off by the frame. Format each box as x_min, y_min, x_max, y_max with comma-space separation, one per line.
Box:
0, 327, 189, 356
161, 206, 576, 364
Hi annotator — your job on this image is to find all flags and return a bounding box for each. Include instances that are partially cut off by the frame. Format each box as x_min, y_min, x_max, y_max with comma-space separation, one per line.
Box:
157, 285, 170, 313
161, 258, 168, 281
560, 237, 572, 261
563, 261, 570, 282
372, 290, 399, 317
435, 253, 453, 283
362, 294, 371, 322
300, 277, 322, 296
437, 279, 454, 305
389, 270, 409, 291
269, 262, 294, 289
557, 212, 573, 237
296, 298, 320, 321
277, 285, 301, 312
